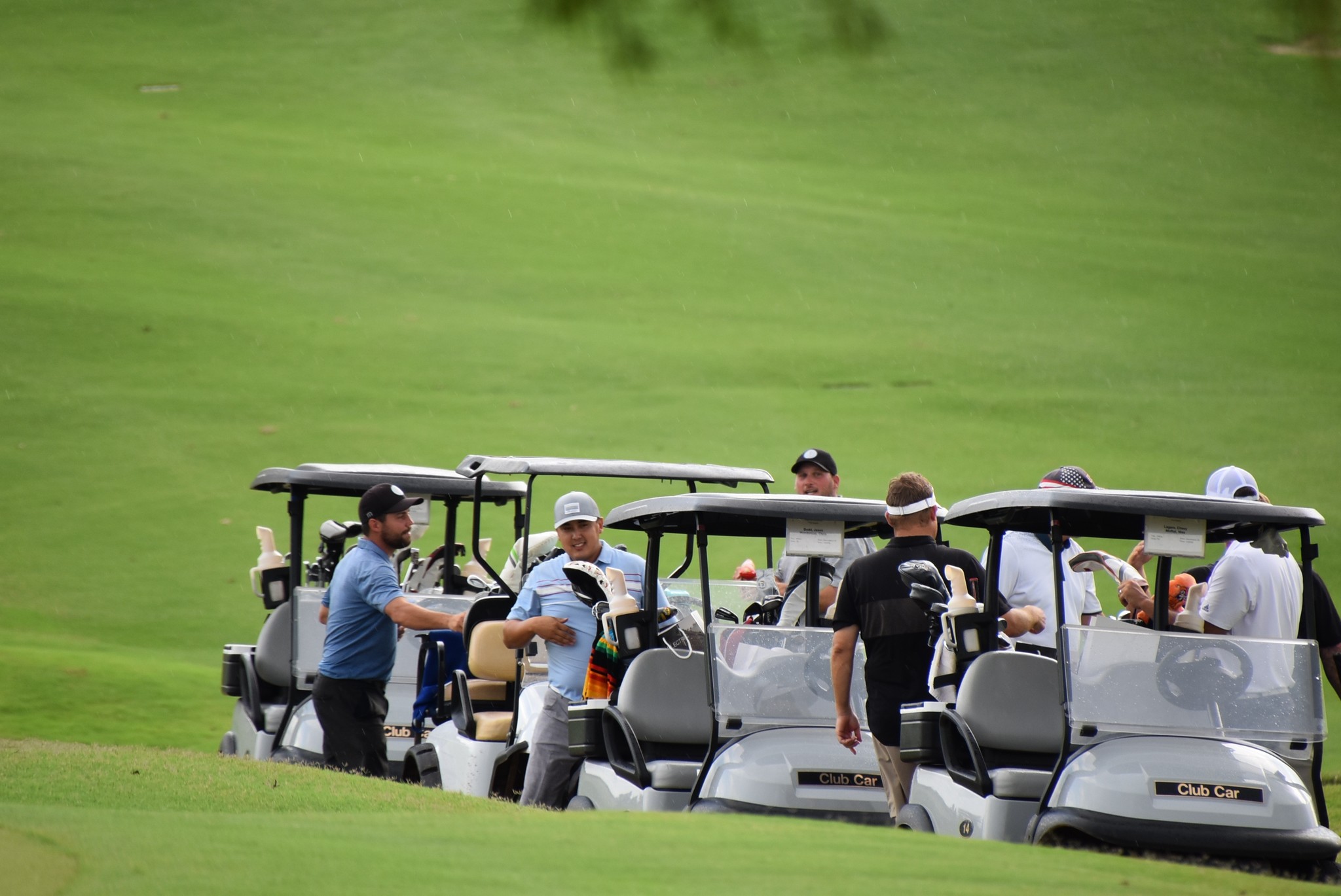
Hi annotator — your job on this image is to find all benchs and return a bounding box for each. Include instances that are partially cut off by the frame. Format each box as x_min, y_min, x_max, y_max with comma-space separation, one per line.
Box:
939, 651, 1211, 800
239, 602, 297, 733
450, 619, 548, 741
603, 649, 836, 790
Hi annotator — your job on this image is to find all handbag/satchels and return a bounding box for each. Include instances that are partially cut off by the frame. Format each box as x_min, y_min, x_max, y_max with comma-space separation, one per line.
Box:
582, 606, 693, 702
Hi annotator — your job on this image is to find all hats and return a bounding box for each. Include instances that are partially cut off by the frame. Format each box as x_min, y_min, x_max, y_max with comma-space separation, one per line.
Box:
554, 491, 601, 529
791, 449, 837, 476
358, 483, 424, 525
1205, 466, 1260, 501
1038, 465, 1108, 491
886, 484, 942, 516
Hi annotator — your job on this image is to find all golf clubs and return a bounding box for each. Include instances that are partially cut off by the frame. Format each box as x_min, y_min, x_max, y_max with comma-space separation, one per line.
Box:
433, 565, 461, 589
743, 594, 783, 625
302, 556, 334, 588
467, 575, 490, 591
394, 547, 425, 592
715, 607, 739, 624
970, 578, 979, 602
998, 634, 1012, 650
592, 601, 609, 620
998, 617, 1007, 632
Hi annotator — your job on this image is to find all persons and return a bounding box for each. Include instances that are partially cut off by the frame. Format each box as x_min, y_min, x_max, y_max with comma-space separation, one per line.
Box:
832, 471, 1046, 824
312, 482, 468, 779
502, 492, 680, 810
775, 449, 876, 626
981, 465, 1341, 830
